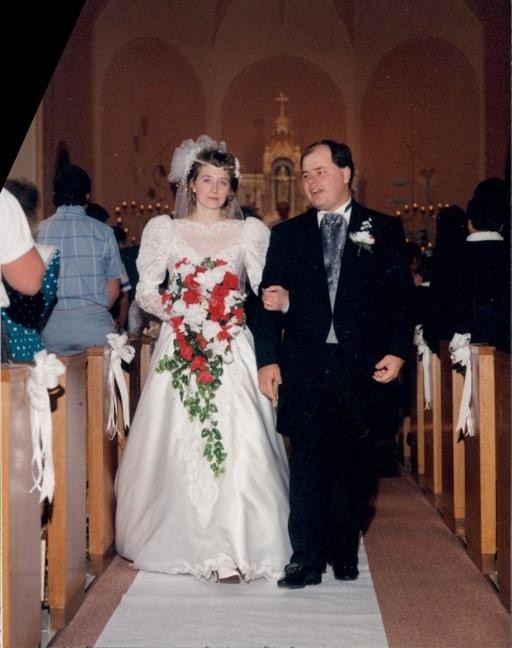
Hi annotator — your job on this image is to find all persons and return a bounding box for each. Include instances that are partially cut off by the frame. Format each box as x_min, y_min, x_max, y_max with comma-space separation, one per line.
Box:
0, 181, 45, 369
113, 134, 290, 589
38, 169, 139, 356
3, 177, 59, 364
276, 200, 289, 218
254, 139, 416, 588
416, 178, 512, 359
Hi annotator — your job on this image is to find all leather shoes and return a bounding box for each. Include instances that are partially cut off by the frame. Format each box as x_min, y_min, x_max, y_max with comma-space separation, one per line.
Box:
277, 560, 323, 589
332, 562, 359, 580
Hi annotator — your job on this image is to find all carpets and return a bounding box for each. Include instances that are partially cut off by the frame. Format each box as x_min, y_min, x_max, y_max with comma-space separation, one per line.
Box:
95, 531, 389, 648
